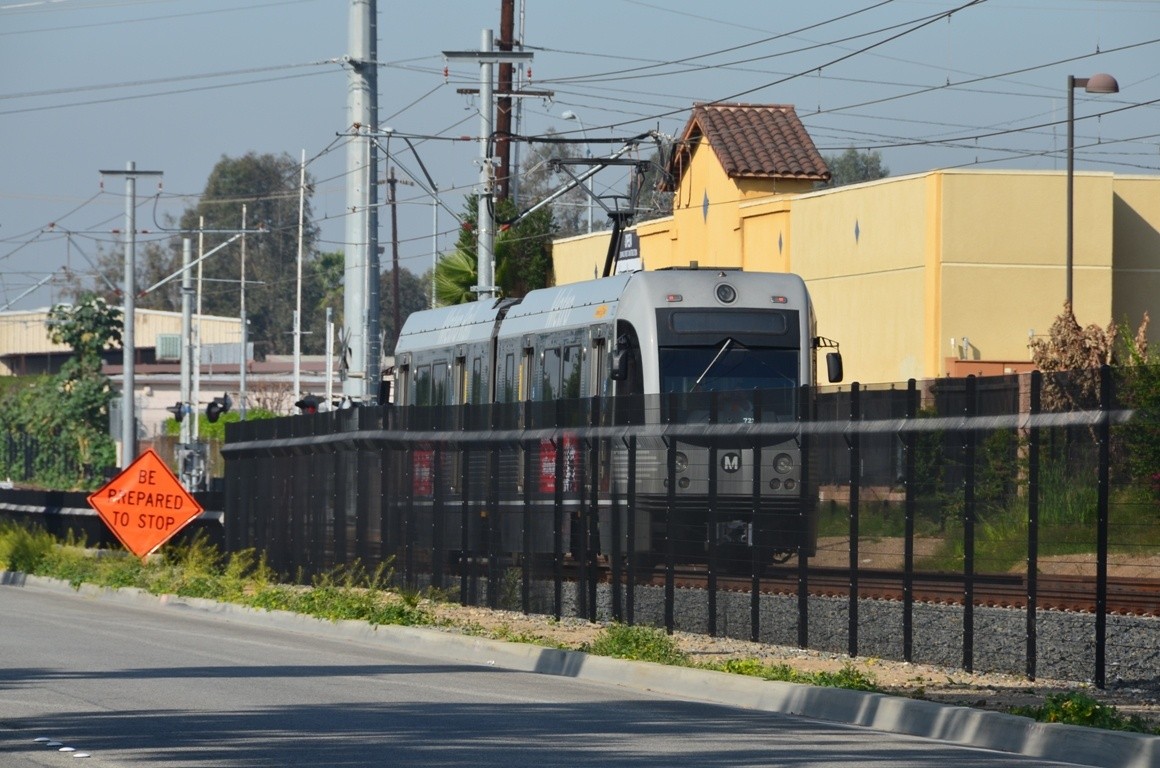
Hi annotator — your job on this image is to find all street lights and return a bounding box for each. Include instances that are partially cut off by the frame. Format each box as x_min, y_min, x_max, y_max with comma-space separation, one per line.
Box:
1063, 73, 1121, 324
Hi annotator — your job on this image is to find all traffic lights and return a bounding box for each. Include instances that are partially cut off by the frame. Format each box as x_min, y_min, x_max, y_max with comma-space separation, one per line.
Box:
206, 402, 224, 423
294, 394, 320, 416
214, 392, 233, 414
166, 401, 187, 422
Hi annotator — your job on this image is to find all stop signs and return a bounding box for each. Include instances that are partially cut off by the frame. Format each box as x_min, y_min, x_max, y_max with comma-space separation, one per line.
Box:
85, 449, 205, 564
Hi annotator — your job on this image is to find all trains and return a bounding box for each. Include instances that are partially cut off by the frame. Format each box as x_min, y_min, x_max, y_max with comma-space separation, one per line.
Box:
388, 262, 843, 575
